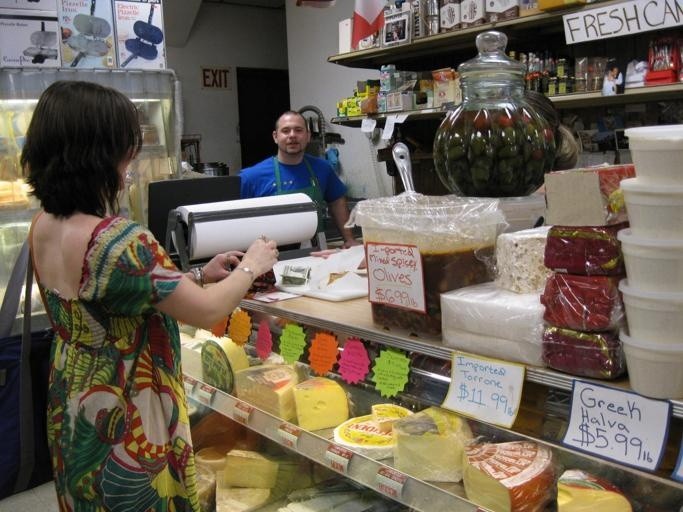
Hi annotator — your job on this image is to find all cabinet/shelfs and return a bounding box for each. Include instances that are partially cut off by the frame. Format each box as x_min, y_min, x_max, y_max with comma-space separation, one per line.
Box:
168, 274, 682, 510
330, 0, 682, 149
0, 67, 181, 505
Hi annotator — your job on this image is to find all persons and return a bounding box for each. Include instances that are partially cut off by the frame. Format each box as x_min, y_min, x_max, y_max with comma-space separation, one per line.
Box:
603, 62, 619, 95
601, 105, 618, 129
17, 79, 279, 511
237, 109, 360, 251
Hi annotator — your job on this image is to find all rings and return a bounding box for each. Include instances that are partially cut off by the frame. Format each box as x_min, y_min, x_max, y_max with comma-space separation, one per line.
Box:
223, 254, 231, 266
257, 233, 268, 242
272, 248, 280, 258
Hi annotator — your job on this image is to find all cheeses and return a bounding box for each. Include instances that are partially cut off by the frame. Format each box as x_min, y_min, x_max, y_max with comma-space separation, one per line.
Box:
460, 438, 558, 512
201, 335, 250, 395
391, 406, 474, 484
495, 232, 553, 294
291, 374, 350, 432
555, 468, 633, 512
233, 363, 297, 420
333, 401, 417, 461
181, 329, 221, 384
185, 411, 406, 512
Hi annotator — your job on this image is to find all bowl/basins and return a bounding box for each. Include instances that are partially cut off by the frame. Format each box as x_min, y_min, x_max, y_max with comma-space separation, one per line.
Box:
618, 121, 683, 406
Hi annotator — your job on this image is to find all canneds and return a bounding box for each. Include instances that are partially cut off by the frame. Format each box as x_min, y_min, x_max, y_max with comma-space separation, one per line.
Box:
547, 58, 602, 96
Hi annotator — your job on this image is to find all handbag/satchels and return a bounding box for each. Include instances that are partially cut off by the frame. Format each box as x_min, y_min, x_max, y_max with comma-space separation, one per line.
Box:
0, 324, 53, 493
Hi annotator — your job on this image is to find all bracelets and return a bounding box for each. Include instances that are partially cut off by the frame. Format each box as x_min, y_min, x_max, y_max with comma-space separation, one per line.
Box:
341, 233, 355, 244
188, 267, 205, 288
235, 263, 254, 285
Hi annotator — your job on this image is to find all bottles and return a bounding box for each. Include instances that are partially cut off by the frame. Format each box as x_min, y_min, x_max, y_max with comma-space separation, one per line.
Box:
650, 46, 674, 72
504, 46, 552, 78
